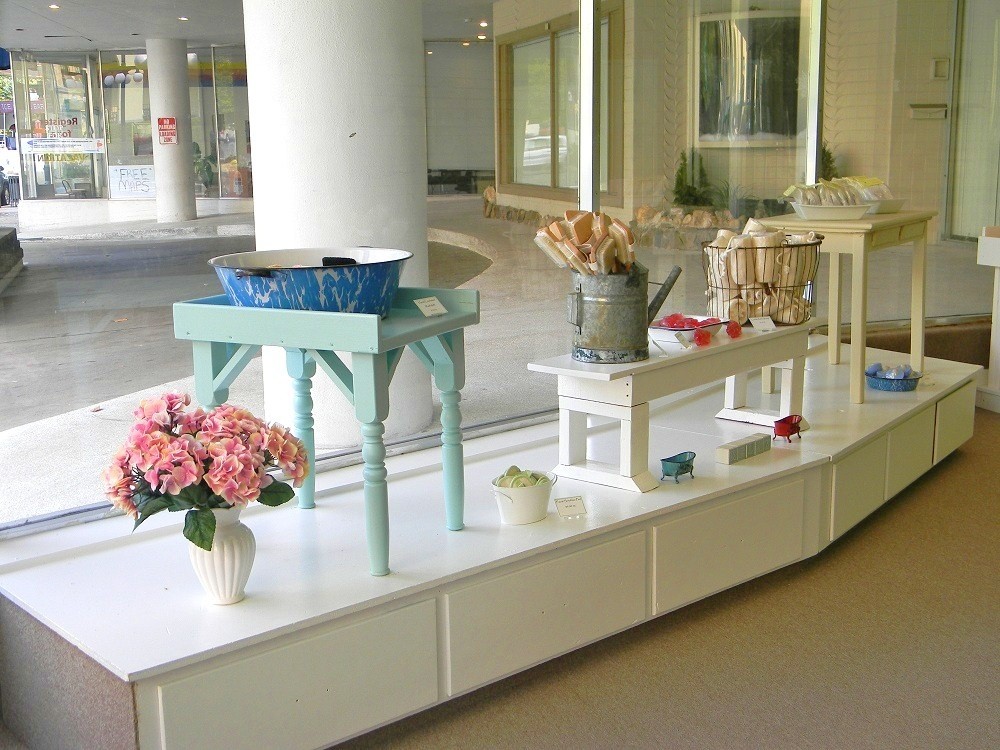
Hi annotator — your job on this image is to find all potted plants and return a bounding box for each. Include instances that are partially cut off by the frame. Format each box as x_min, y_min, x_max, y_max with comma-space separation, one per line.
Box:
671, 149, 760, 227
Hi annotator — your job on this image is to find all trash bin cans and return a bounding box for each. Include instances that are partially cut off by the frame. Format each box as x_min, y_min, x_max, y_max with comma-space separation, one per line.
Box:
7, 175, 20, 207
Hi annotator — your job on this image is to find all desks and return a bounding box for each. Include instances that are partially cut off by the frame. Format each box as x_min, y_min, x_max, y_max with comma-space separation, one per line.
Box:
527, 316, 825, 494
757, 209, 937, 403
172, 286, 480, 577
72, 178, 92, 199
37, 184, 55, 199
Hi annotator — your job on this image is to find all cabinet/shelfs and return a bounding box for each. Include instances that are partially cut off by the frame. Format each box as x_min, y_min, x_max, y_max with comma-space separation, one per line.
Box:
0, 415, 829, 750
648, 343, 983, 551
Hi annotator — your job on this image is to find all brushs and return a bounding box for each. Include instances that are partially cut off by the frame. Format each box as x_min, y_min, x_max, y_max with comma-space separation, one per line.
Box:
533, 209, 637, 277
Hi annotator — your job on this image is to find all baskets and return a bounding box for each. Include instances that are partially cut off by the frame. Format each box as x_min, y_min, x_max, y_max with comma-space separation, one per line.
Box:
701, 233, 825, 326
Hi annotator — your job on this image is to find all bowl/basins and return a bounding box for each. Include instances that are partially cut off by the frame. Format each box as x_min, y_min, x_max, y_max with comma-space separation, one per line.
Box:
863, 198, 906, 214
789, 201, 870, 220
206, 245, 413, 320
647, 314, 730, 343
865, 372, 923, 391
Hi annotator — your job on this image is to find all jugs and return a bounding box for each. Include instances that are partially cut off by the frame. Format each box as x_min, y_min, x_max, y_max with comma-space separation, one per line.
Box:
566, 265, 682, 363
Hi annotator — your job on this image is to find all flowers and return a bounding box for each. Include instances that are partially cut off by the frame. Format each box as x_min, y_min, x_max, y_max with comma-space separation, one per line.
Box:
103, 389, 309, 551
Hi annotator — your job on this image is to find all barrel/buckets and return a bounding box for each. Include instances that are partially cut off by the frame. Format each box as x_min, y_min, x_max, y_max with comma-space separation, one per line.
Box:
491, 471, 557, 525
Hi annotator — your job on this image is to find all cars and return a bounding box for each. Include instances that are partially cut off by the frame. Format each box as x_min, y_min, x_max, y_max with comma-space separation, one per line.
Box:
0, 165, 12, 207
521, 134, 567, 166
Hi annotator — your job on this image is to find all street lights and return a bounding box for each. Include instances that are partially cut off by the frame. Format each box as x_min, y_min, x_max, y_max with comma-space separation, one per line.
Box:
102, 53, 143, 165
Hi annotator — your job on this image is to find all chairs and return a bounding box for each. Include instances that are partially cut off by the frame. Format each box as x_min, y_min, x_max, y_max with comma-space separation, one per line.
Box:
62, 180, 88, 199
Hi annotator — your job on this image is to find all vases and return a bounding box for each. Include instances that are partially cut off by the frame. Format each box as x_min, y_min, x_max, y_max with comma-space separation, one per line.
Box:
763, 199, 788, 218
187, 504, 255, 605
